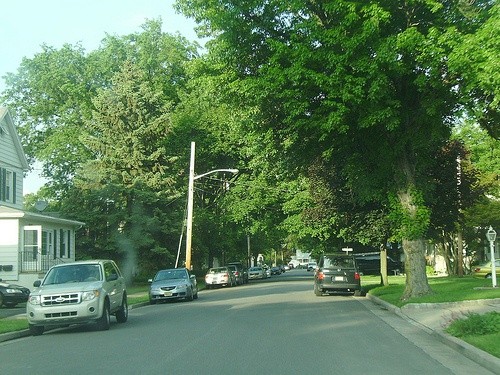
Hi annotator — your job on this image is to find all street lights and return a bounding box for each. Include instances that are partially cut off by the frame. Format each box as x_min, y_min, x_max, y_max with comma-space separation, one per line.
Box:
185, 142, 240, 271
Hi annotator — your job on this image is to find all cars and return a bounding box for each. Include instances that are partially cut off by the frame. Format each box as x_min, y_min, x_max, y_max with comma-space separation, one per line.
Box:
262, 262, 317, 279
148, 267, 198, 304
247, 267, 267, 280
227, 262, 248, 286
204, 266, 236, 289
486, 225, 497, 288
0, 278, 31, 309
471, 258, 500, 280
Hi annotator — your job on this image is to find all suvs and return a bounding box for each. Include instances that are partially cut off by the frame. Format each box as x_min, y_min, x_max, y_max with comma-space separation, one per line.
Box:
309, 252, 361, 297
357, 252, 405, 276
26, 259, 128, 336
